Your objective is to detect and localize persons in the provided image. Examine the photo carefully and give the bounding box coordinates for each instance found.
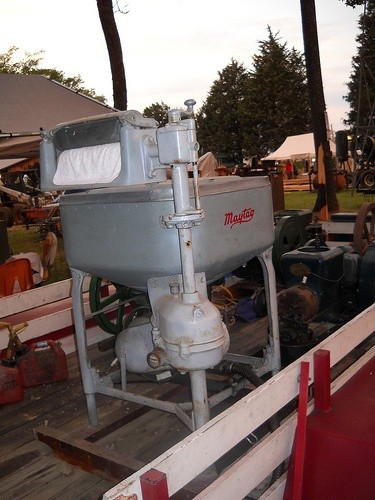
[285,157,311,179]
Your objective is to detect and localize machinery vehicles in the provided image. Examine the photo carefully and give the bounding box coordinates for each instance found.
[0,168,61,226]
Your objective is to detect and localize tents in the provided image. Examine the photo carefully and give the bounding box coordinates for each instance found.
[261,132,353,179]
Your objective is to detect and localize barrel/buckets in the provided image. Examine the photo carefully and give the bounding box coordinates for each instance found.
[0,321,69,405]
